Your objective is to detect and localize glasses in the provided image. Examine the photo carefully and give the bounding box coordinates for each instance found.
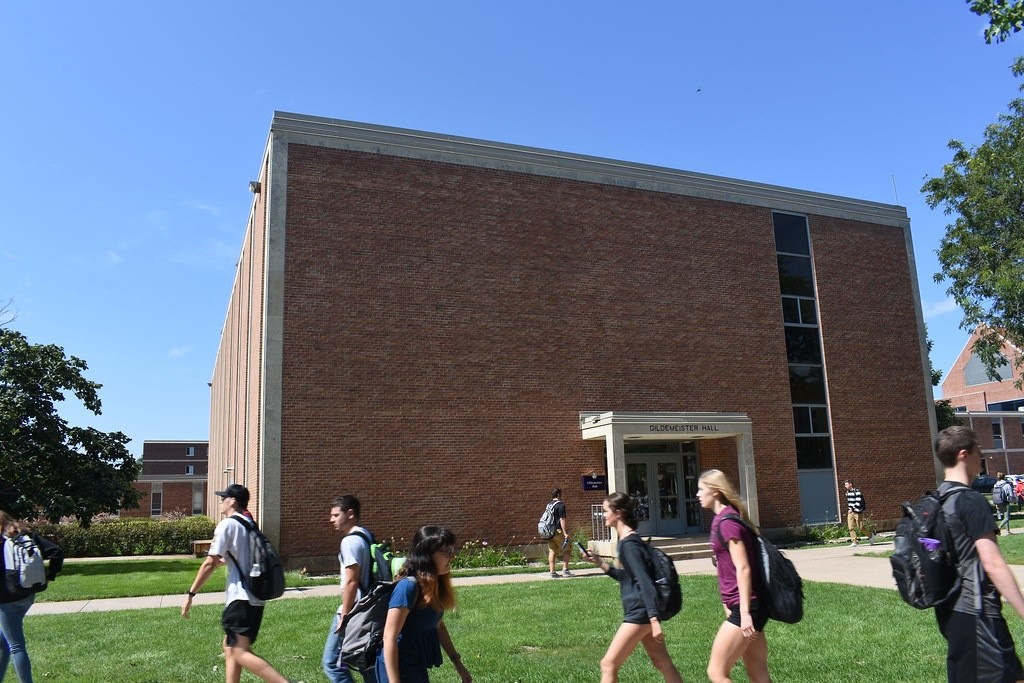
[437,548,454,557]
[221,496,227,500]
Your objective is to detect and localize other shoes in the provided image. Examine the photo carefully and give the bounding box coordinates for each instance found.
[870,532,874,545]
[1006,531,1011,534]
[849,542,857,547]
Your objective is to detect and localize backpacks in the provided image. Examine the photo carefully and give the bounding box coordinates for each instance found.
[2,530,48,593]
[616,538,683,620]
[226,515,285,600]
[340,531,408,593]
[333,576,422,672]
[717,513,803,624]
[992,482,1009,505]
[890,486,979,609]
[537,500,565,539]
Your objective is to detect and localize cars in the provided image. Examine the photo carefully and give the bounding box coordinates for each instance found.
[972,476,998,494]
[1004,474,1024,486]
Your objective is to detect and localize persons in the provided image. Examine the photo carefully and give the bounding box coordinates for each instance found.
[182,484,288,683]
[0,509,64,683]
[579,492,683,683]
[375,527,472,683]
[322,495,375,683]
[934,425,1024,683]
[697,469,773,683]
[1014,478,1024,512]
[546,488,576,577]
[844,479,874,548]
[994,471,1013,536]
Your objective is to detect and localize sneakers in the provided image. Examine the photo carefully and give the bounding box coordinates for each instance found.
[560,570,575,577]
[549,572,563,578]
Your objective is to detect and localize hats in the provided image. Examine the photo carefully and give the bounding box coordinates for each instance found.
[215,484,249,502]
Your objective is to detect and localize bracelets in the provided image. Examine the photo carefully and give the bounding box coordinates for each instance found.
[450,654,461,662]
[187,589,195,596]
